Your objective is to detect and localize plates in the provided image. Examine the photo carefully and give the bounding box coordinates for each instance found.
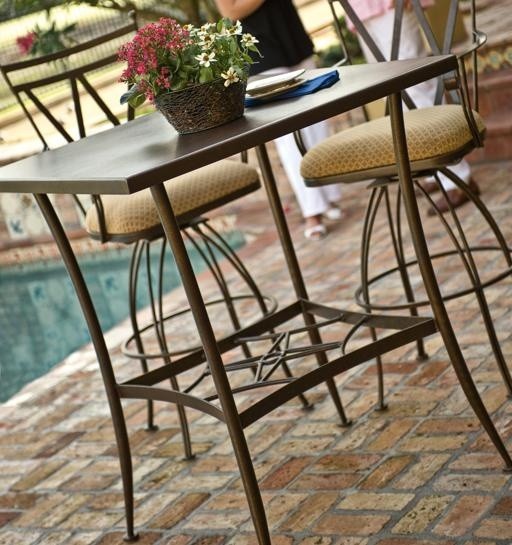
[246,68,308,103]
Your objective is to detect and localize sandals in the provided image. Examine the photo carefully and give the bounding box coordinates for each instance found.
[304,206,344,241]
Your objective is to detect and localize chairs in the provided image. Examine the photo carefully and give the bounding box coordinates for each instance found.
[0,8,315,461]
[294,0,511,412]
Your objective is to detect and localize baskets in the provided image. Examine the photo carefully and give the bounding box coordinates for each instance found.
[154,66,247,135]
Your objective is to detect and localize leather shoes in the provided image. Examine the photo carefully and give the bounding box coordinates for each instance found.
[415,177,480,216]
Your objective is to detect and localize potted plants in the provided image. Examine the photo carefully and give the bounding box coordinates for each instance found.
[115,15,264,135]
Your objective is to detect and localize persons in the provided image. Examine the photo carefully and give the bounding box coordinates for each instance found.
[345,0,481,216]
[213,0,348,240]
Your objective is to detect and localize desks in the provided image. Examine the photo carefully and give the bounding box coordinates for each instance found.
[2,52,512,545]
